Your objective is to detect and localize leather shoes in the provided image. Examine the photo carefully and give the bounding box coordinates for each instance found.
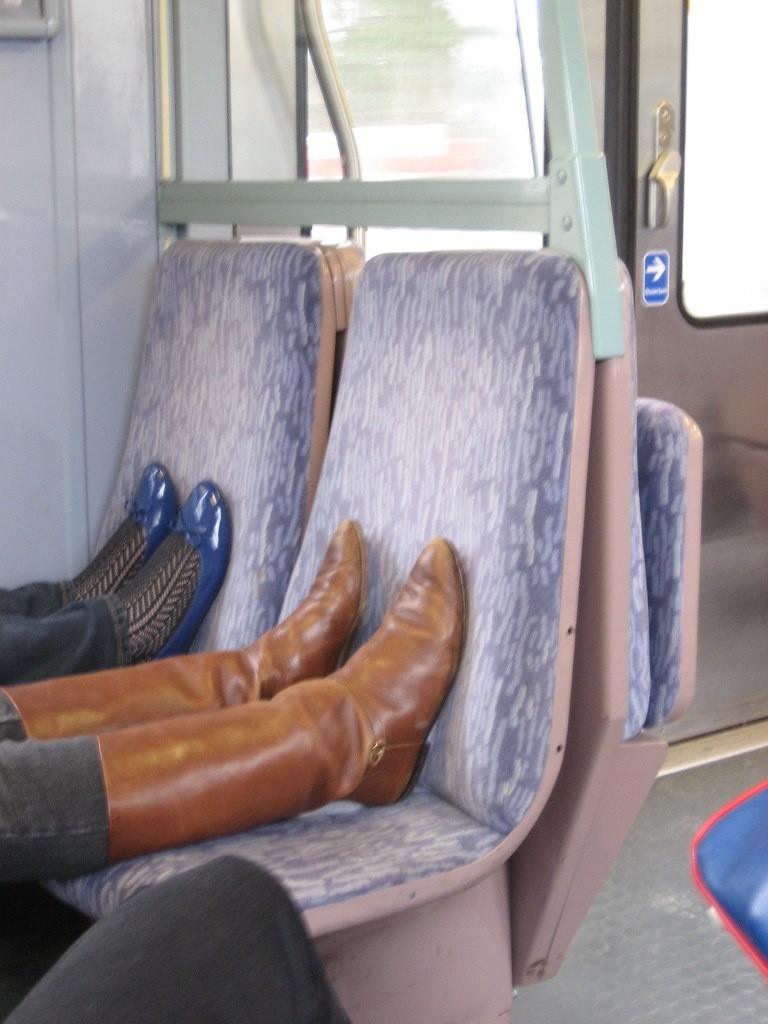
[122,461,179,568]
[155,481,233,660]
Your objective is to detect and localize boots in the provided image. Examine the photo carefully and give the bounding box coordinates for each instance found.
[0,520,367,745]
[97,537,468,866]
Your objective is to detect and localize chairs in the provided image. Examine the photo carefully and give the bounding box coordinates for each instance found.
[40,236,702,1024]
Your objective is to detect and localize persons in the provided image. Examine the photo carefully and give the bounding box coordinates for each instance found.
[0,518,466,884]
[0,463,232,688]
